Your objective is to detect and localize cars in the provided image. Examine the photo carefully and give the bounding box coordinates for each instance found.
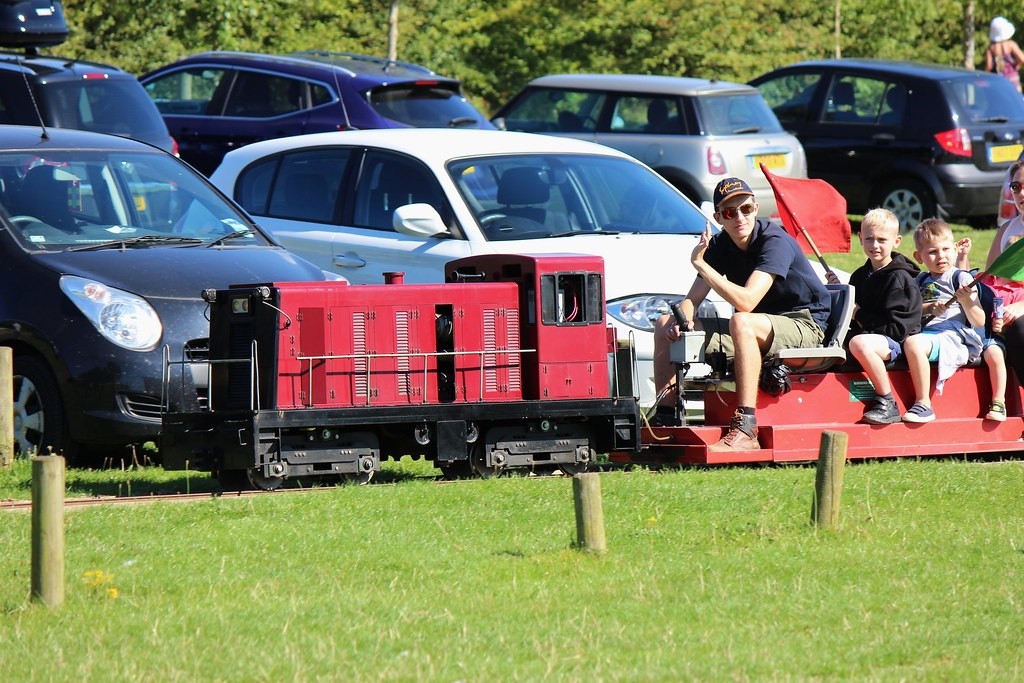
[742,60,1024,233]
[481,72,814,227]
[175,127,856,449]
[0,124,352,460]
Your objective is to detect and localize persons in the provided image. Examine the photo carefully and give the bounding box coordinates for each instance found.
[646,177,832,451]
[825,148,1024,423]
[987,16,1024,94]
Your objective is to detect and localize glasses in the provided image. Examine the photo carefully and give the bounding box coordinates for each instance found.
[1009,181,1024,194]
[718,203,754,220]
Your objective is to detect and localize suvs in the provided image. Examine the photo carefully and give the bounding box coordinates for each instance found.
[0,0,181,233]
[126,51,500,229]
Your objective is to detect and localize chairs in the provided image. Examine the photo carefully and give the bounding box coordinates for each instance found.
[643,102,669,132]
[368,161,428,230]
[281,173,333,223]
[769,283,853,370]
[497,168,556,223]
[876,87,907,126]
[830,81,858,122]
[16,164,78,232]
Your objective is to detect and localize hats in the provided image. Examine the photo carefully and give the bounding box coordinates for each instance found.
[990,17,1016,42]
[713,177,754,208]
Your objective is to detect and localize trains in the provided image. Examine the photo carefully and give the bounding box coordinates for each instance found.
[156,255,1020,492]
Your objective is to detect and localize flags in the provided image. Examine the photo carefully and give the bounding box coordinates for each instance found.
[761,163,852,254]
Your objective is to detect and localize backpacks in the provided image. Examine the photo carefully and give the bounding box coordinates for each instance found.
[919,269,999,339]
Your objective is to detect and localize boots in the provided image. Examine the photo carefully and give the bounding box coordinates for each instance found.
[645,412,682,426]
[708,408,761,451]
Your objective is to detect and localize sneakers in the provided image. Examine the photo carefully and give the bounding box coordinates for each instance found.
[862,396,901,423]
[986,399,1006,422]
[901,403,936,422]
